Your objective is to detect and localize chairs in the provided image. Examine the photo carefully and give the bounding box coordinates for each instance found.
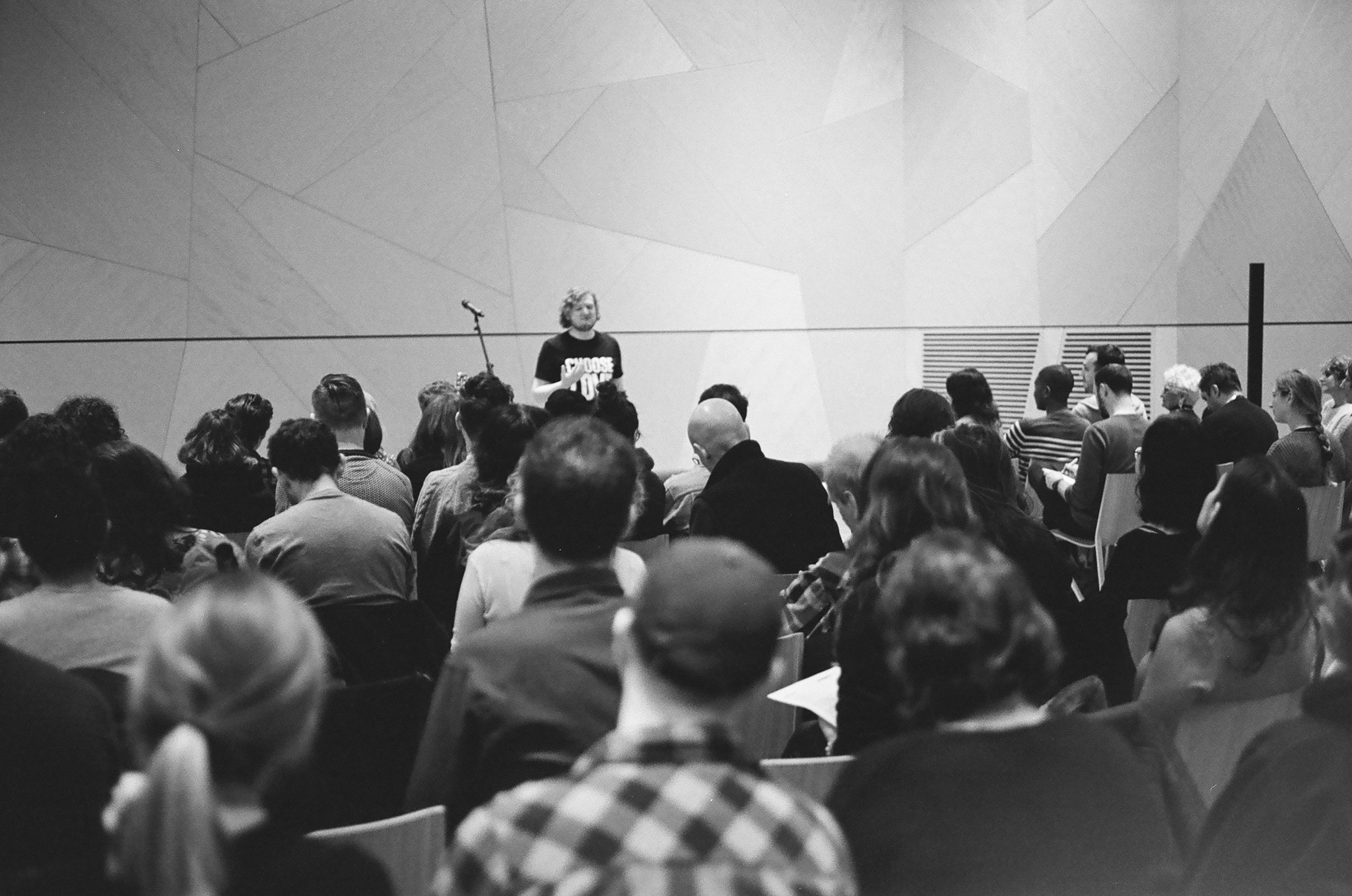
[1298,479,1347,568]
[1189,712,1352,896]
[1046,470,1149,592]
[293,593,424,802]
[733,633,804,763]
[758,753,858,804]
[613,534,668,578]
[1165,683,1311,815]
[305,804,450,894]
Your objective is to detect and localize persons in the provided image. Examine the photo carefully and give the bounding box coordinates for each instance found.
[801,345,1350,665]
[683,397,839,580]
[0,455,178,677]
[92,570,395,896]
[777,435,985,708]
[424,536,854,896]
[528,288,624,399]
[96,439,218,602]
[405,413,630,827]
[1133,455,1325,748]
[3,373,753,550]
[1174,523,1352,896]
[244,415,419,605]
[822,524,1184,896]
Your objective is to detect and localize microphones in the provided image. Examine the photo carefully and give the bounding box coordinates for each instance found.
[462,300,485,318]
